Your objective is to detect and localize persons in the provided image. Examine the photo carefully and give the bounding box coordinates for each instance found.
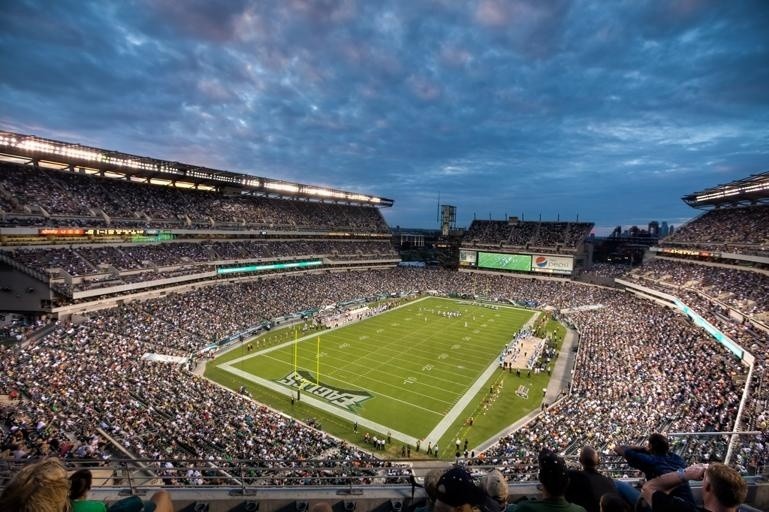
[0,161,769,512]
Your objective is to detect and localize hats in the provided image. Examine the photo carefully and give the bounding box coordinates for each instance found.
[424,461,510,510]
